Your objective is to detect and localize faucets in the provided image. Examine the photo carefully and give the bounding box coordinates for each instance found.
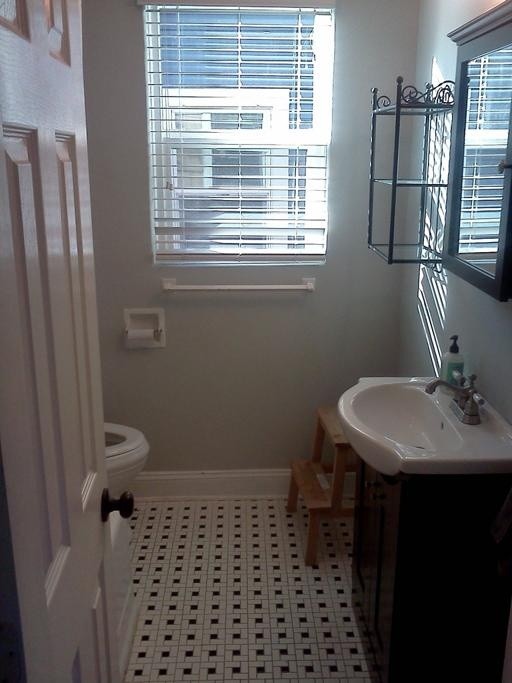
[423,378,468,396]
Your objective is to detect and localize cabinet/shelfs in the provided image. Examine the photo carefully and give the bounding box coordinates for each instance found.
[366,74,455,274]
[350,455,511,683]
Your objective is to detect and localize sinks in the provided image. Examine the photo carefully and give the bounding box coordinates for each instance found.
[335,375,512,473]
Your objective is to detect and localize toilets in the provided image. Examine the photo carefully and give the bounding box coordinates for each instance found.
[103,425,150,492]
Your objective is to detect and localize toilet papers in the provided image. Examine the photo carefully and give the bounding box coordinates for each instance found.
[126,329,155,341]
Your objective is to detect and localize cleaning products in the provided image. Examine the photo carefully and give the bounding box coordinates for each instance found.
[435,336,465,388]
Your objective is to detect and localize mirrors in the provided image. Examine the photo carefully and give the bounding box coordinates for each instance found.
[440,0,511,302]
